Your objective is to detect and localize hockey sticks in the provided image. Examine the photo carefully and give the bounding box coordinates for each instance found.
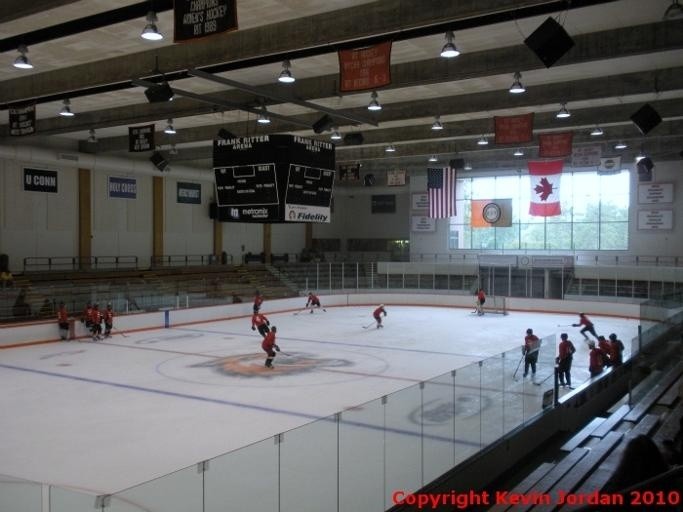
[533,372,553,386]
[513,354,525,382]
[362,314,384,329]
[470,308,477,313]
[113,325,130,338]
[293,306,308,316]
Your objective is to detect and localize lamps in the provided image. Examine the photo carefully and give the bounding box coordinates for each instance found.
[7,8,651,169]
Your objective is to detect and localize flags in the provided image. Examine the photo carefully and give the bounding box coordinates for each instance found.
[471,199,513,227]
[424,167,457,217]
[527,160,561,216]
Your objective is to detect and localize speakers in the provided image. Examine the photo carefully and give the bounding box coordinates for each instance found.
[629,104,662,136]
[144,84,174,103]
[639,157,654,173]
[344,134,364,145]
[523,17,574,68]
[312,114,333,134]
[449,159,464,168]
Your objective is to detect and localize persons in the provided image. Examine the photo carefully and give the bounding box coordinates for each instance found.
[251,308,279,354]
[372,304,388,329]
[607,333,624,367]
[586,341,609,378]
[0,270,13,287]
[522,329,539,379]
[572,312,600,340]
[475,288,486,315]
[596,336,612,366]
[305,292,327,314]
[12,289,113,342]
[261,326,278,368]
[555,333,576,386]
[252,290,264,313]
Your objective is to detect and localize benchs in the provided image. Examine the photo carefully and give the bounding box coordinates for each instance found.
[398,269,682,303]
[0,250,396,327]
[475,345,682,512]
